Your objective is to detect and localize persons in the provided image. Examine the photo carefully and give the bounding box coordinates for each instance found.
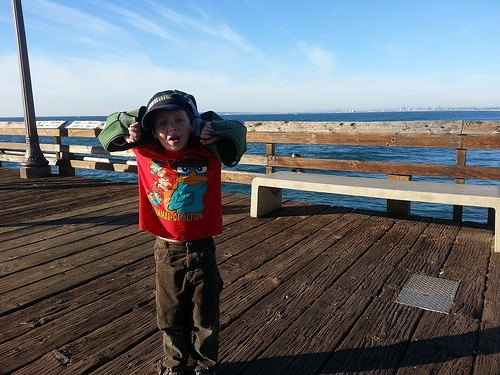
[291,152,305,173]
[99,91,249,375]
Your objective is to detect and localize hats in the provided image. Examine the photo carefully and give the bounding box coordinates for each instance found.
[141,91,188,130]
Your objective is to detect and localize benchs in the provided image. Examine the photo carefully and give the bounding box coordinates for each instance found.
[250,171,499,251]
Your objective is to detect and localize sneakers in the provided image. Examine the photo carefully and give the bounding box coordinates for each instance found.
[195,368,216,375]
[161,367,184,375]
